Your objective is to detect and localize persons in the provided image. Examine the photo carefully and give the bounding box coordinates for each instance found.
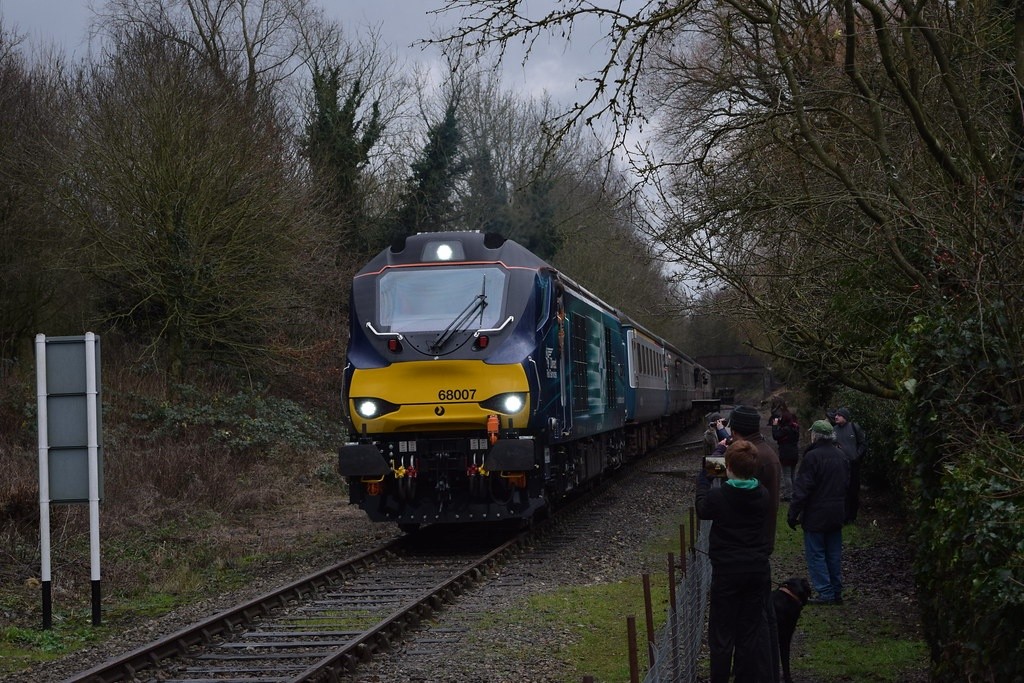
[788,420,852,607]
[709,407,783,602]
[789,413,798,428]
[823,409,838,426]
[832,409,867,523]
[699,408,733,461]
[769,409,799,501]
[692,439,778,683]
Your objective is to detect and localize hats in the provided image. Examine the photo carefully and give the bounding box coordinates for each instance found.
[808,420,833,434]
[728,406,761,432]
[834,408,851,419]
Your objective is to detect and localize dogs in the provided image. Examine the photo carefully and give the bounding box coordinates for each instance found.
[771,577,811,683]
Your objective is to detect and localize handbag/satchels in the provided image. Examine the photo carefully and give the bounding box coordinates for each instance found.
[861,449,867,462]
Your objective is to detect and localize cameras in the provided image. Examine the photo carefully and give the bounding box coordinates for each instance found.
[709,418,727,428]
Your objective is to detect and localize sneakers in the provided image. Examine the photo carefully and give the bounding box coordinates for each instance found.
[808,592,832,605]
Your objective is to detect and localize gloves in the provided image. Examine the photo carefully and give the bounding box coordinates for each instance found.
[699,469,716,488]
[787,517,800,531]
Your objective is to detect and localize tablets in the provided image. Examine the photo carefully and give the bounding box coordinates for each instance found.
[703,455,733,480]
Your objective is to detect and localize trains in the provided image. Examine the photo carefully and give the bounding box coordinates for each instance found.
[717,385,736,403]
[336,229,717,546]
